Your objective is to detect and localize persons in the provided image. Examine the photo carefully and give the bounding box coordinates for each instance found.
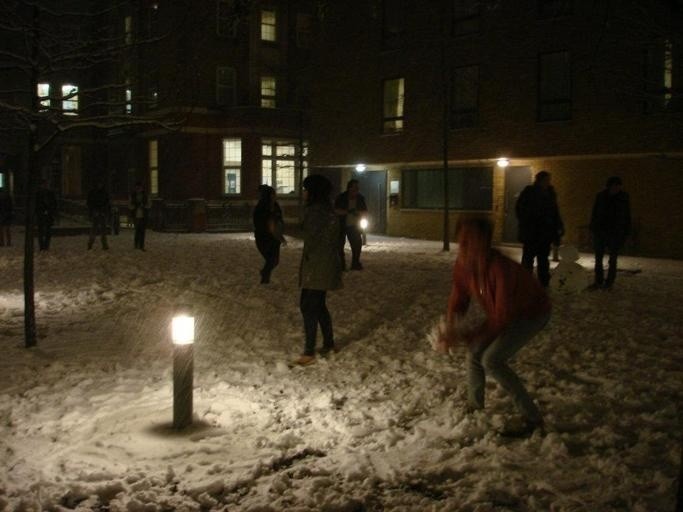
[128,180,153,252]
[0,186,12,250]
[584,176,632,292]
[84,176,110,252]
[252,183,288,284]
[34,177,55,252]
[271,174,345,365]
[514,169,565,287]
[336,180,369,271]
[430,213,551,440]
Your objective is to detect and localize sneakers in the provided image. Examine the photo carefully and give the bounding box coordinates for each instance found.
[497,421,544,439]
[297,353,316,366]
